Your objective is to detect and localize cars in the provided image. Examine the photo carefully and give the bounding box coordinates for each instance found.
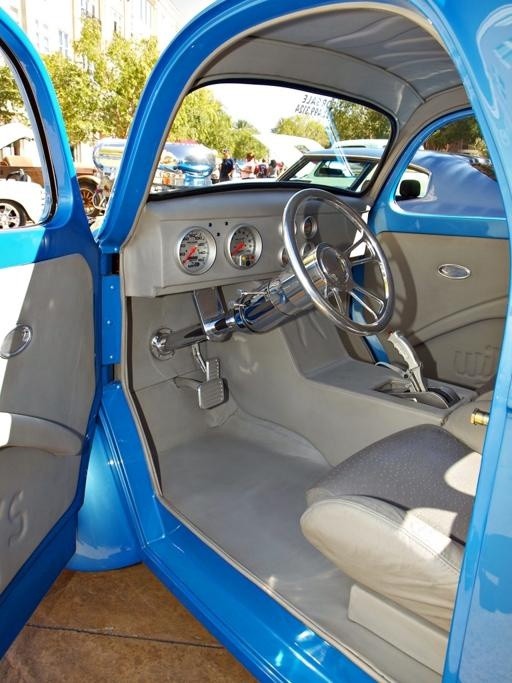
[0,122,45,228]
[296,137,427,199]
[2,0,510,683]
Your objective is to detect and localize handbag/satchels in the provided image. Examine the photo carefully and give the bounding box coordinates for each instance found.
[254,166,260,175]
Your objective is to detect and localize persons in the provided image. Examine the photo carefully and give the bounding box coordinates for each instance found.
[218,147,236,183]
[256,158,270,177]
[266,158,278,178]
[210,149,223,184]
[238,151,259,178]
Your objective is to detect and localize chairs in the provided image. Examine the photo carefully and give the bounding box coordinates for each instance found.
[299,400,500,676]
